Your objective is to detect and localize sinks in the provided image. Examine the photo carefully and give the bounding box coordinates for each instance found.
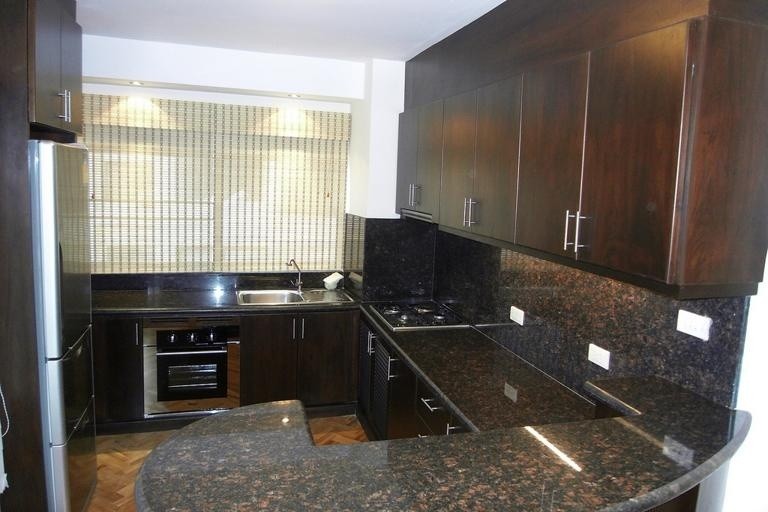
[236,289,305,306]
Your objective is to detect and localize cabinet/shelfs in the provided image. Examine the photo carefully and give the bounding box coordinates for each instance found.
[441,75,523,245]
[91,313,145,421]
[385,352,414,441]
[517,17,768,286]
[417,378,453,439]
[394,99,439,224]
[241,309,355,403]
[357,322,393,440]
[2,0,85,140]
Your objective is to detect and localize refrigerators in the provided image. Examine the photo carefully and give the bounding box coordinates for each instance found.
[29,138,98,512]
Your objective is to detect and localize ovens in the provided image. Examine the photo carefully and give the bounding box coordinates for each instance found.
[142,319,242,419]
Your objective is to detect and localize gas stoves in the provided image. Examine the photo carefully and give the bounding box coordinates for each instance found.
[369,299,470,330]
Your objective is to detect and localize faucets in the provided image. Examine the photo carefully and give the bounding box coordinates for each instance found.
[285,258,304,288]
